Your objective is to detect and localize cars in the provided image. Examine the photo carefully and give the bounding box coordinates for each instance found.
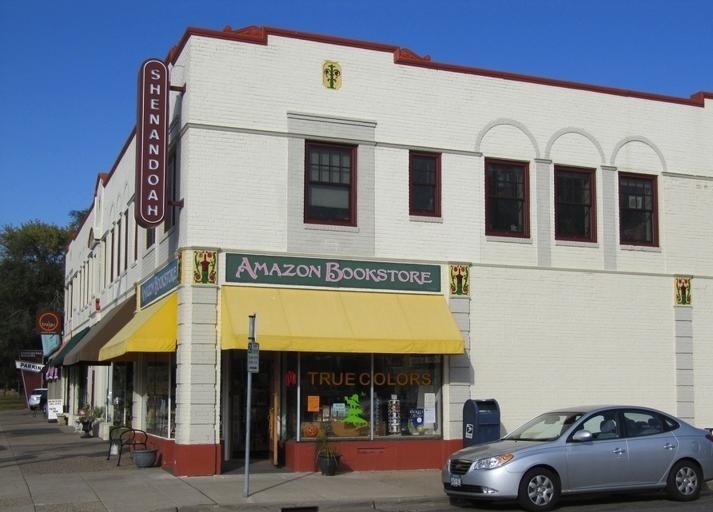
[317,395,423,421]
[442,399,712,512]
[28,388,48,410]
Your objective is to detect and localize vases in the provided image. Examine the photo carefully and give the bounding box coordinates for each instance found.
[56,412,65,425]
[133,447,157,467]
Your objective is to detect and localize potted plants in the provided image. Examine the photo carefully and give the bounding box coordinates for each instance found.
[75,401,103,438]
[313,424,343,476]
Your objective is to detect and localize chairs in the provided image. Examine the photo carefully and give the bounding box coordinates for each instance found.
[115,428,148,466]
[107,425,135,460]
